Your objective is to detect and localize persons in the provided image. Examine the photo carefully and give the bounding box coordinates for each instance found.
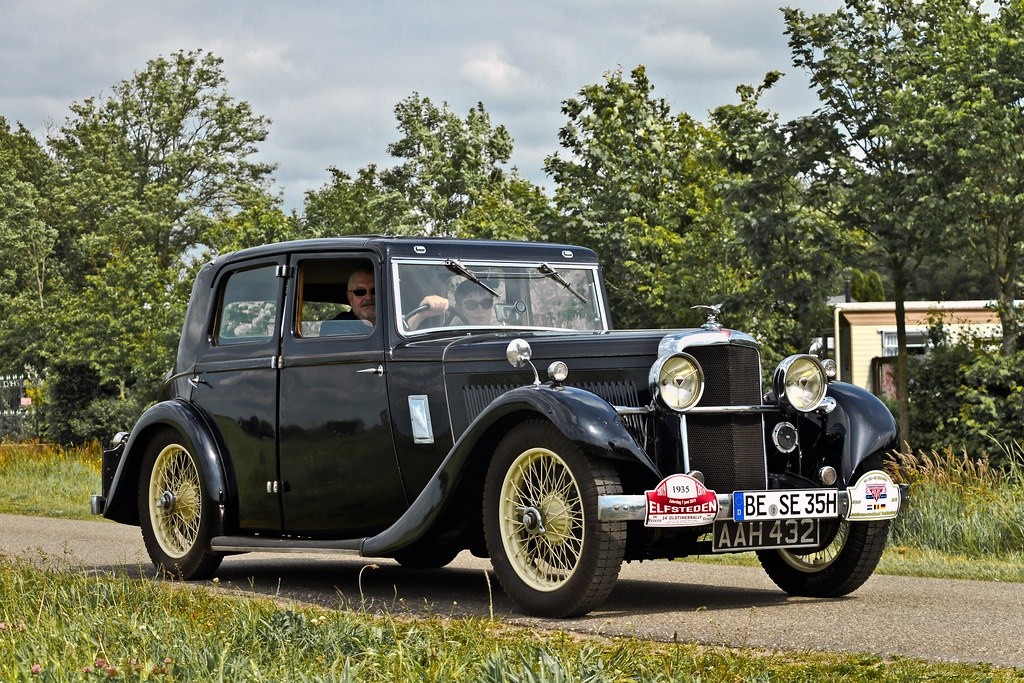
[318,270,449,337]
[455,279,495,325]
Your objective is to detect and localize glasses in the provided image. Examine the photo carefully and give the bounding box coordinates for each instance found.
[348,286,375,296]
[459,297,493,311]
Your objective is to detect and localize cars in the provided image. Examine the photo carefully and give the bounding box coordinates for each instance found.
[88,233,912,622]
